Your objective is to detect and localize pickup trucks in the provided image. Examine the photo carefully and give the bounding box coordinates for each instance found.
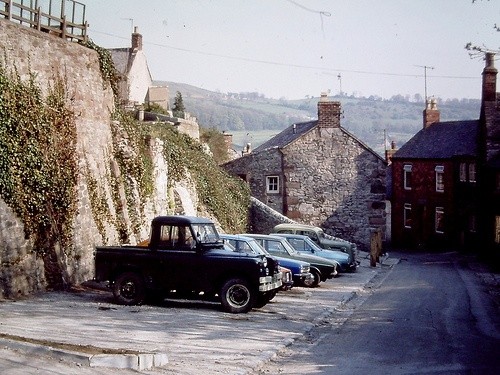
[94,215,284,314]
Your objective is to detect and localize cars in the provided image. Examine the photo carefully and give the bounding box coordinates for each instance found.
[200,234,311,290]
[268,233,350,278]
[238,234,337,288]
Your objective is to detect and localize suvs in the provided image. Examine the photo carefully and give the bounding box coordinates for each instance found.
[272,223,361,273]
